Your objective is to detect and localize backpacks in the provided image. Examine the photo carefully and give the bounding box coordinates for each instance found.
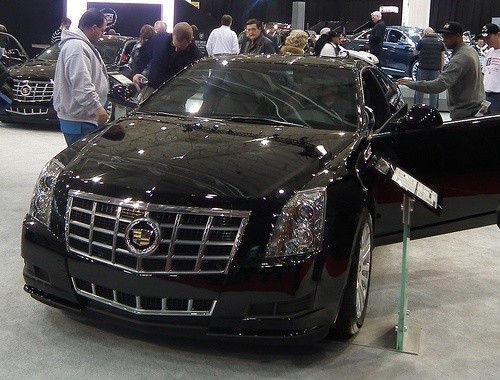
[314,36,335,56]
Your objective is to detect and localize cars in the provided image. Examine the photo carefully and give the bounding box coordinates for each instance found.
[349,25,450,82]
[0,31,138,128]
[20,52,499,349]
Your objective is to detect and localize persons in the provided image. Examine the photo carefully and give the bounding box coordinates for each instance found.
[52,7,111,147]
[394,21,485,121]
[470,23,500,114]
[0,10,500,108]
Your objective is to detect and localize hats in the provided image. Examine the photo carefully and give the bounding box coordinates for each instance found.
[478,24,500,37]
[437,22,465,34]
[328,31,339,37]
[320,27,331,36]
